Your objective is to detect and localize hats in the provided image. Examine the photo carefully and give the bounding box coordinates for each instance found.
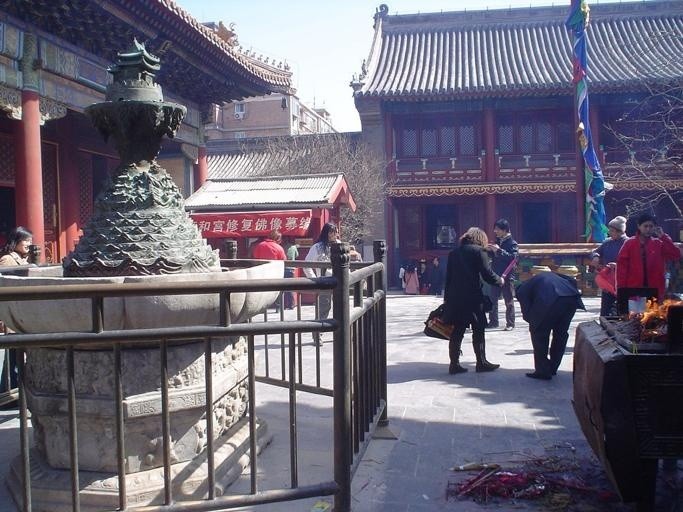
[607,216,628,233]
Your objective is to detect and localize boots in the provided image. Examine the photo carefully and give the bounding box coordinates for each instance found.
[447,343,468,374]
[473,341,500,373]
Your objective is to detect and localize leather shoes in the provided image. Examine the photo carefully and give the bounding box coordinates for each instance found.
[487,324,498,328]
[524,370,557,381]
[505,325,514,330]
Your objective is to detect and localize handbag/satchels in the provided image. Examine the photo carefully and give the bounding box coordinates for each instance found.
[423,303,458,340]
[596,262,618,297]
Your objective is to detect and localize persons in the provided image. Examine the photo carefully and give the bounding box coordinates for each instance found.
[252,231,288,261]
[485,219,519,331]
[282,237,299,310]
[443,226,505,374]
[302,222,340,346]
[350,245,366,297]
[398,256,443,297]
[590,214,683,317]
[1,225,33,407]
[514,273,586,381]
